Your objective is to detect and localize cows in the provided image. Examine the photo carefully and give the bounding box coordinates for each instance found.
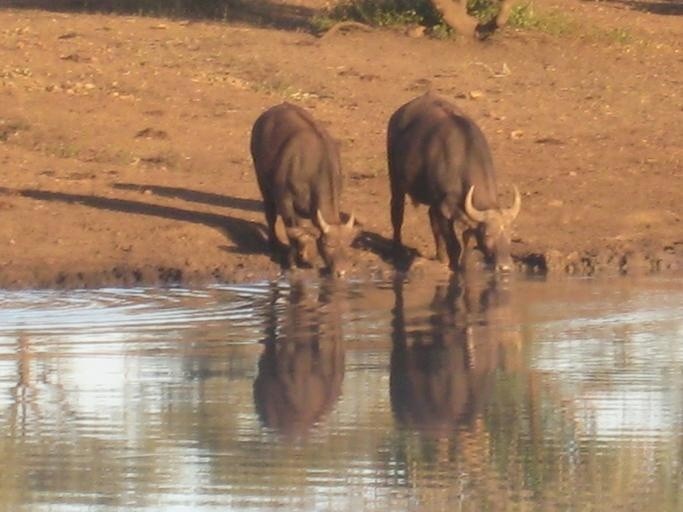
[249,101,355,280]
[386,92,520,275]
